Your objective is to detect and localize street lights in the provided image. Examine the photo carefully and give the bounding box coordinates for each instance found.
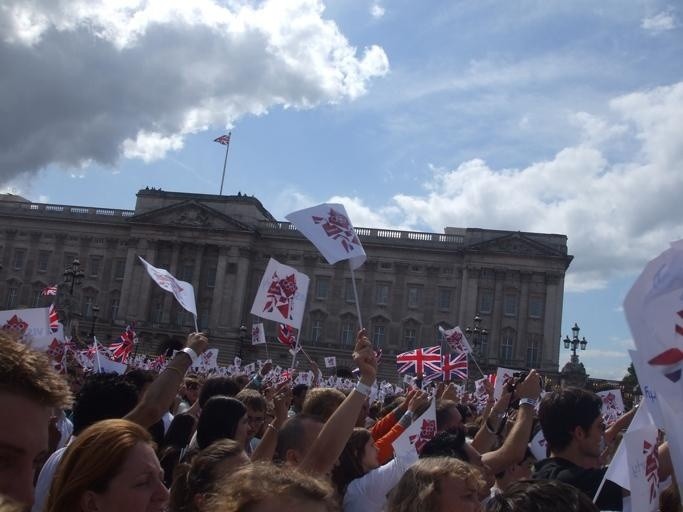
[465,312,487,377]
[62,257,84,296]
[89,303,100,337]
[562,321,587,356]
[237,319,247,362]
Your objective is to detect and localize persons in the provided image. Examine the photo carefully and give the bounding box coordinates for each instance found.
[1,323,681,512]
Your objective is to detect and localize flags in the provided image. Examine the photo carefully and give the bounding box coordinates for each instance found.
[40,284,57,296]
[47,302,63,312]
[250,258,312,331]
[623,238,683,416]
[285,202,367,273]
[139,257,199,321]
[214,135,231,145]
[47,305,59,329]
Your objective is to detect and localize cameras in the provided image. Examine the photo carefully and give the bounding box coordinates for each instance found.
[507,371,544,410]
